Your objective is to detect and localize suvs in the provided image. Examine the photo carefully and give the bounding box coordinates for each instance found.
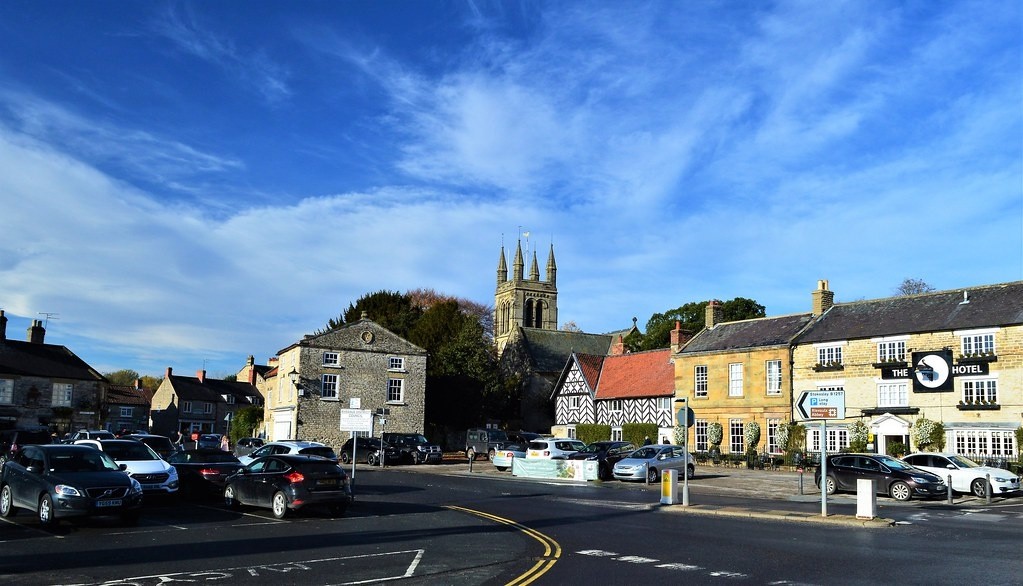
[817,453,947,503]
[380,431,442,465]
[466,428,524,463]
[568,441,636,481]
[527,438,589,461]
[236,441,338,469]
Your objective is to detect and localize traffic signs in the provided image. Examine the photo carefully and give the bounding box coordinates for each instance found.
[795,390,846,421]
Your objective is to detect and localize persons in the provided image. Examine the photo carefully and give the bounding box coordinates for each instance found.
[3,427,236,465]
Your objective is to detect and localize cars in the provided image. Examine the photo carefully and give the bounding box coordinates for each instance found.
[492,434,542,471]
[898,452,1020,499]
[1,424,248,527]
[224,453,352,519]
[613,444,695,485]
[340,436,400,466]
